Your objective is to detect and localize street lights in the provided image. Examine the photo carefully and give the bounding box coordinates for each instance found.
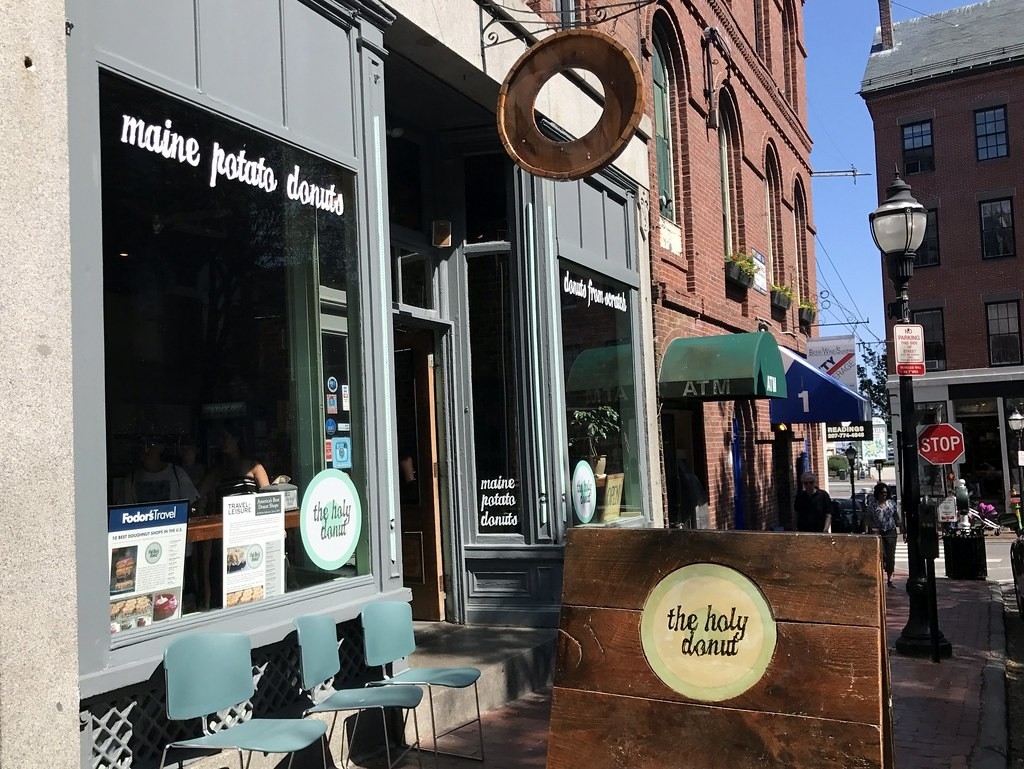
[845,443,857,532]
[1008,406,1024,526]
[874,459,884,483]
[868,163,953,652]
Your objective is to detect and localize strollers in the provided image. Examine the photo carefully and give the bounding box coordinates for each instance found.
[968,502,1002,536]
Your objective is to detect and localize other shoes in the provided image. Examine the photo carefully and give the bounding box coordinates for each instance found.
[887,581,896,588]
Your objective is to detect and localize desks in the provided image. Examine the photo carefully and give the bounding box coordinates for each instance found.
[184,510,299,610]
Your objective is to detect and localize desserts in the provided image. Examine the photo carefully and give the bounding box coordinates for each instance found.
[227,547,246,572]
[110,558,177,634]
[227,586,263,606]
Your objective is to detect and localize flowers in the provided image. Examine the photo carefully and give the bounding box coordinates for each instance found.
[798,302,817,312]
[724,252,760,278]
[773,284,796,306]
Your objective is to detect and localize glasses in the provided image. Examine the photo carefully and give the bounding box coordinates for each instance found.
[802,481,813,486]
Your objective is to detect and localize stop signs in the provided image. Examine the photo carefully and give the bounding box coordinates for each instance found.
[917,423,966,465]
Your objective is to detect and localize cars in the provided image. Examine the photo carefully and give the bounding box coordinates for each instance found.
[832,498,866,529]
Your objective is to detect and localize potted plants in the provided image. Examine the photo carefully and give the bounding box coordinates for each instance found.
[568,406,621,478]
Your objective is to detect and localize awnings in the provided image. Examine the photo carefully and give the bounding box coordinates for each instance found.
[768,346,872,424]
[659,331,790,400]
[568,345,633,405]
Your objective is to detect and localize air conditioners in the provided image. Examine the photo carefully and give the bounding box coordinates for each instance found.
[926,361,946,371]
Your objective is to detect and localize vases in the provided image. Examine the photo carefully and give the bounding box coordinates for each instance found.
[799,308,812,323]
[771,291,791,310]
[725,262,753,288]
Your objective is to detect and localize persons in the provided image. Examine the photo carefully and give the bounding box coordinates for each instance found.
[862,483,901,588]
[792,471,833,533]
[109,425,271,612]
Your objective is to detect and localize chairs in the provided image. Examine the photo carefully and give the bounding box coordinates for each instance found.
[290,614,423,769]
[160,634,328,769]
[345,602,484,769]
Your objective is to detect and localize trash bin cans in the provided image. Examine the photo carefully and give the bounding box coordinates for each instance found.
[941,524,987,581]
